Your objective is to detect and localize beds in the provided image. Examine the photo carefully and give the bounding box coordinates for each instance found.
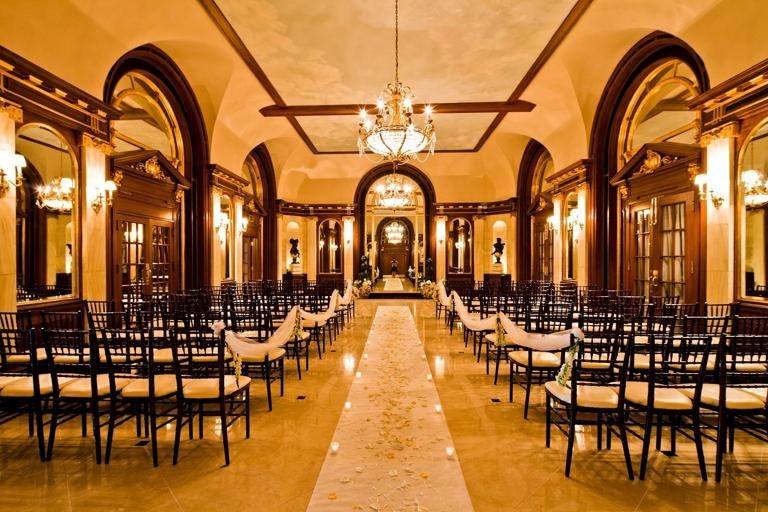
[2,276,357,383]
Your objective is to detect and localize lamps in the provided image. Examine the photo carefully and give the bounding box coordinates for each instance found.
[214,213,228,232]
[567,212,584,243]
[545,215,559,235]
[0,149,26,196]
[691,171,725,209]
[89,180,116,215]
[355,0,438,172]
[740,170,766,207]
[368,173,417,213]
[237,217,247,236]
[33,142,73,213]
[383,222,405,244]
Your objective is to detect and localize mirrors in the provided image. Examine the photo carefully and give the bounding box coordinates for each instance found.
[14,111,80,305]
[353,162,437,299]
[559,192,576,279]
[375,217,414,279]
[445,215,472,274]
[317,216,344,275]
[734,112,766,308]
[217,192,236,281]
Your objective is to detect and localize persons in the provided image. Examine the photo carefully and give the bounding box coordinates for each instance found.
[390,257,400,278]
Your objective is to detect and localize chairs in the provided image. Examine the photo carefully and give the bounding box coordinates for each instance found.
[432,278,763,388]
[608,334,713,483]
[670,334,766,485]
[99,326,191,469]
[223,310,287,412]
[167,325,253,466]
[1,373,35,438]
[39,326,138,465]
[1,328,82,462]
[508,314,573,420]
[543,332,637,481]
